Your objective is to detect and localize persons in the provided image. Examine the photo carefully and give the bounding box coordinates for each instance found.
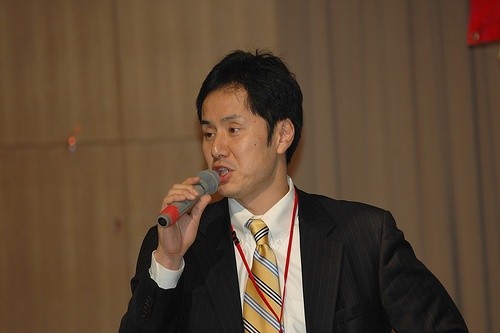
[118,47,469,333]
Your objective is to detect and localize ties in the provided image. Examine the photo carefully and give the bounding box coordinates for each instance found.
[242,219,285,333]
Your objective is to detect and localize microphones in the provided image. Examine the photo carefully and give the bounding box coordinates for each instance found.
[157,169,221,228]
